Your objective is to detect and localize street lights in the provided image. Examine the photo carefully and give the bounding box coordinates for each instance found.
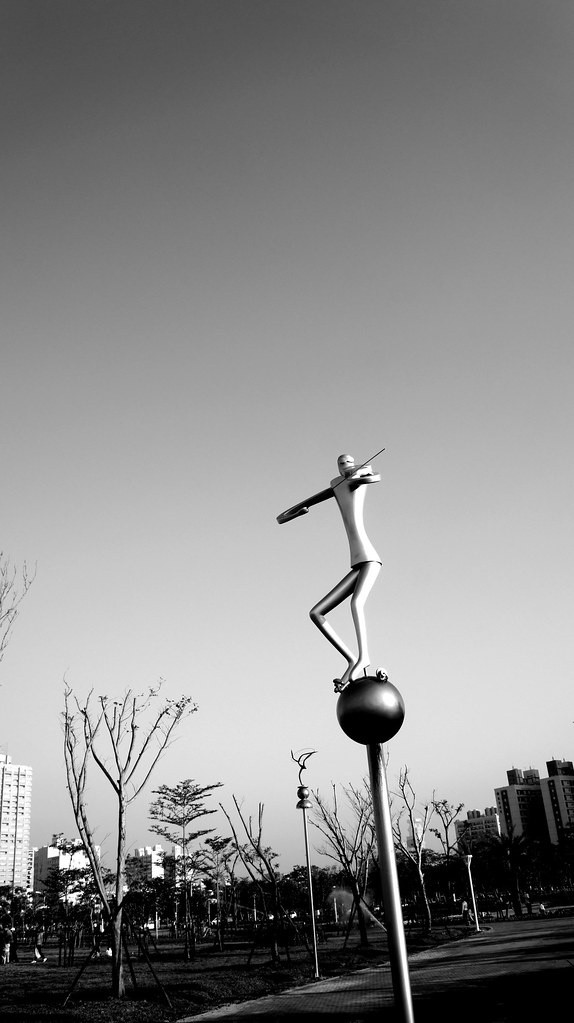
[463,854,481,931]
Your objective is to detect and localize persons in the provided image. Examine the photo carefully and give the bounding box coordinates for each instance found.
[31,927,47,964]
[0,925,19,964]
[524,890,533,915]
[461,898,470,926]
[539,902,545,914]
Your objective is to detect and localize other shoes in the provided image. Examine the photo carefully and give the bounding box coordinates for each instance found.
[31,960,36,964]
[42,958,47,962]
